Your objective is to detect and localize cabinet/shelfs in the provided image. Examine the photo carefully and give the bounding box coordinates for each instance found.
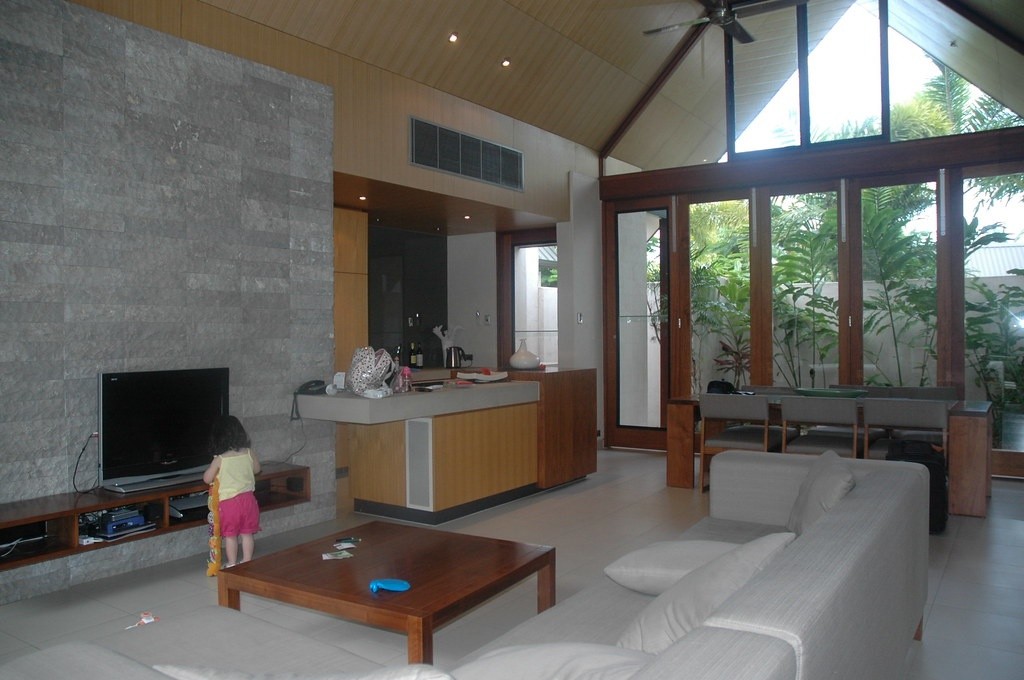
[0,460,311,573]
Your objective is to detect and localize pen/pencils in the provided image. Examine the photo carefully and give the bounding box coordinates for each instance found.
[336,537,362,542]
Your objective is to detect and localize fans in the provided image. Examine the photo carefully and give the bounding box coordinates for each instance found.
[643,0,777,43]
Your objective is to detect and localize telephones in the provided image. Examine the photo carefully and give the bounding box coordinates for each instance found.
[297,379,328,395]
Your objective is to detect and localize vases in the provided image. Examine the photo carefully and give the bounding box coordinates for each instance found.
[509,339,539,370]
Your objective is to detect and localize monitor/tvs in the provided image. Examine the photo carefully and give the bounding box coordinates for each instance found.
[98,366,229,493]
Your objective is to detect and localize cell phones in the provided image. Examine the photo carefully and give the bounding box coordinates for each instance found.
[336,536,362,543]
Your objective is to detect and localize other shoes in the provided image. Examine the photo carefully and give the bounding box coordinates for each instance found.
[222,561,243,569]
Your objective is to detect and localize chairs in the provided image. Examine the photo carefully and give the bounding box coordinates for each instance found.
[888,386,958,442]
[779,394,863,459]
[726,385,802,438]
[806,385,891,442]
[863,398,949,469]
[697,392,782,495]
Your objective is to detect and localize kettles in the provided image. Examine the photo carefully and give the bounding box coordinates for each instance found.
[446,346,466,368]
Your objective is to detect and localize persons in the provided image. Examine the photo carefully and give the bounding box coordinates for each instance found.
[203,416,262,571]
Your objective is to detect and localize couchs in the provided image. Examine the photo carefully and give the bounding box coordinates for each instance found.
[362,449,931,680]
[0,602,386,680]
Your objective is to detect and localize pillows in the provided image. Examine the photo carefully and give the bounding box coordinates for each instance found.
[615,531,796,655]
[450,641,656,680]
[604,540,741,596]
[785,450,856,536]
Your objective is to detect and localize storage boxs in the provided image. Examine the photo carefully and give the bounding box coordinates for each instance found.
[665,392,994,517]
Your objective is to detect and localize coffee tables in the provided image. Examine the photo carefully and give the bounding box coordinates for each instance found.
[216,519,556,666]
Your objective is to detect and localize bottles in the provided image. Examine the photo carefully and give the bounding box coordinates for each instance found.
[416,342,423,369]
[509,339,540,370]
[393,345,400,367]
[409,342,416,368]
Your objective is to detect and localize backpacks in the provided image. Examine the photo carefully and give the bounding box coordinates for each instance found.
[351,346,399,395]
